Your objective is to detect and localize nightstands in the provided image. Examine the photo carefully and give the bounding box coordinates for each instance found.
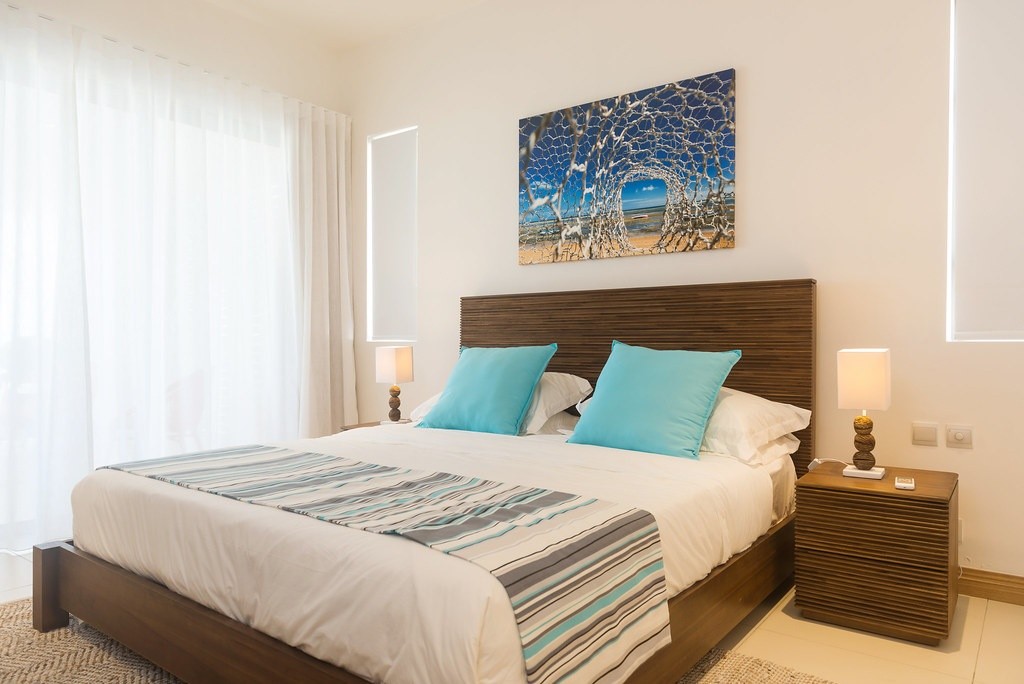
[340,421,402,430]
[794,462,959,648]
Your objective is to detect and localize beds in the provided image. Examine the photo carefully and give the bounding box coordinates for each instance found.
[26,278,817,684]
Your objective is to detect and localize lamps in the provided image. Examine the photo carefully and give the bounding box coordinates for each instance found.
[375,345,412,425]
[837,348,891,479]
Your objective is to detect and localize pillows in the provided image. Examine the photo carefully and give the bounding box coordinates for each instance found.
[565,339,742,458]
[576,387,812,461]
[742,434,801,466]
[414,343,558,434]
[410,371,593,436]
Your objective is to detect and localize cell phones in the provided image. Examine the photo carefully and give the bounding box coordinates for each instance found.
[894,477,915,490]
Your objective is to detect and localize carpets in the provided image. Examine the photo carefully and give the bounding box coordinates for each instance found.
[0,598,833,684]
